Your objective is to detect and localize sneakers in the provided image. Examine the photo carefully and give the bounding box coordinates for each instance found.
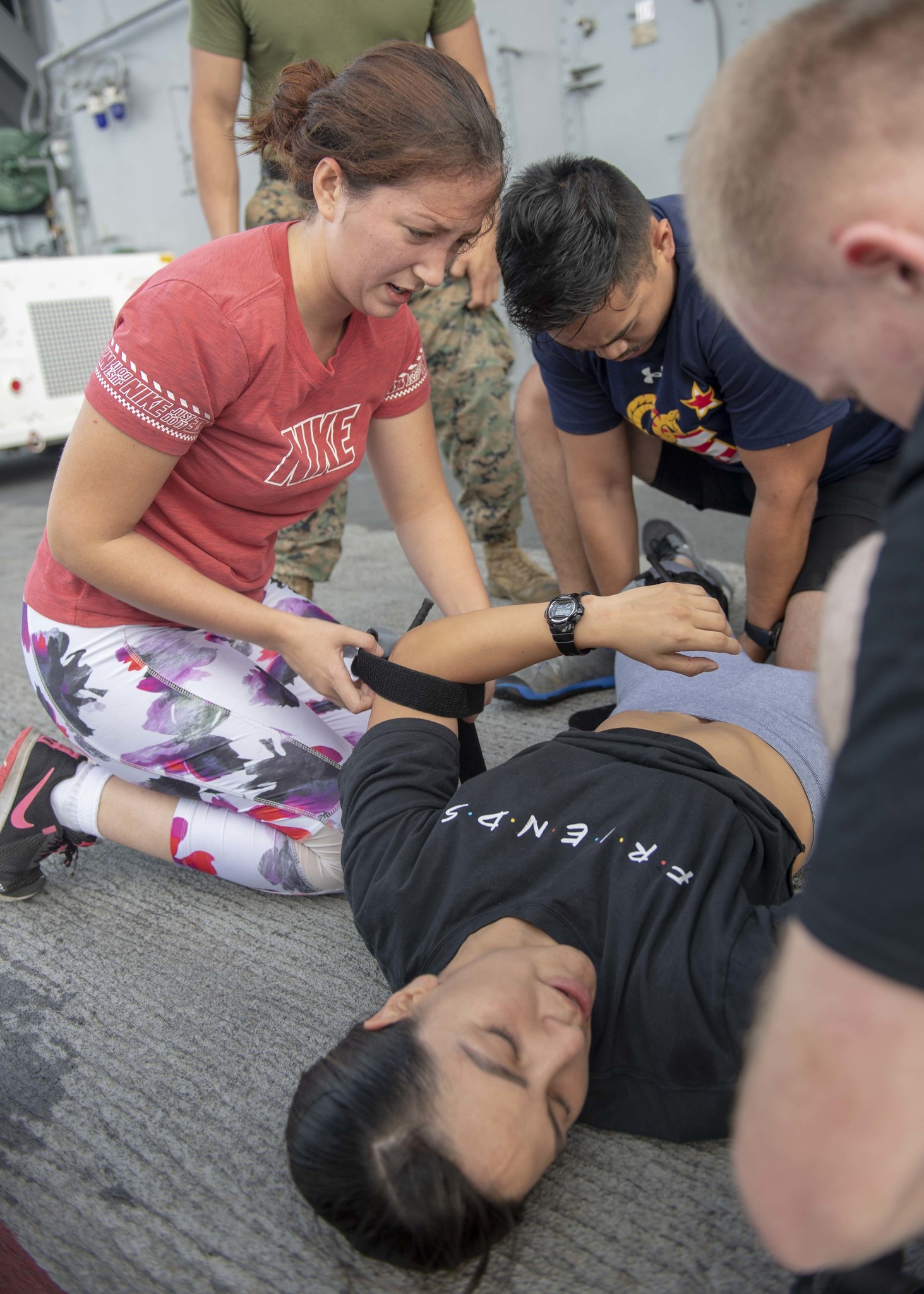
[643,519,735,603]
[0,727,97,902]
[493,645,615,706]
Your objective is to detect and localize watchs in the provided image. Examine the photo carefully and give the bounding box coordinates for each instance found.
[544,590,599,656]
[744,619,784,651]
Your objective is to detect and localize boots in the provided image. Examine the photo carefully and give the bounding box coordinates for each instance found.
[483,529,559,605]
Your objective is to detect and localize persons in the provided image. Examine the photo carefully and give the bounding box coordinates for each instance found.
[495,151,902,703]
[0,42,497,901]
[189,1,564,605]
[679,0,924,1274]
[284,519,836,1272]
